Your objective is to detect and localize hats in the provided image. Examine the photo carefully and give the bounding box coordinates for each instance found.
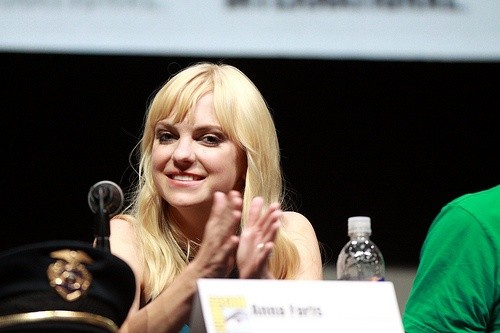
[0,241,137,333]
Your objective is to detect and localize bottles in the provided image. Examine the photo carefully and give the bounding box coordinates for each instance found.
[336,216,385,281]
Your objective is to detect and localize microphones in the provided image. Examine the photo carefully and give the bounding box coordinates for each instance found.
[88,180,124,251]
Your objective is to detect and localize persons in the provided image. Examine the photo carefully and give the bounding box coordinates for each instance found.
[402,185,500,333]
[110,64,322,333]
